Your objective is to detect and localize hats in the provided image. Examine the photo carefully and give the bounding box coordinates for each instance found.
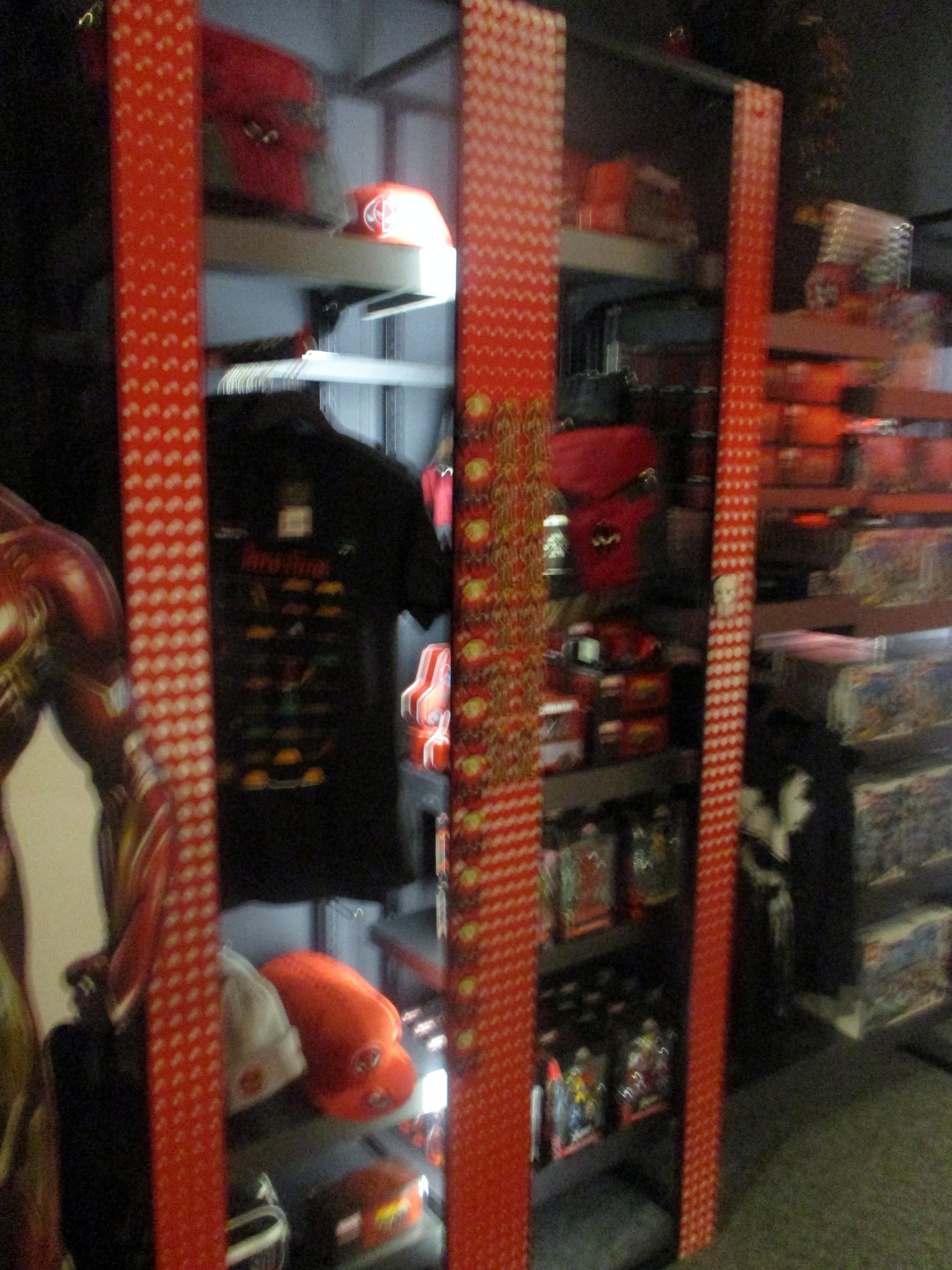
[349,180,455,245]
[222,947,305,1118]
[257,953,416,1122]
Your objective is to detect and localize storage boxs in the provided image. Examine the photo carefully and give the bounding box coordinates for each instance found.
[388,148,951,1165]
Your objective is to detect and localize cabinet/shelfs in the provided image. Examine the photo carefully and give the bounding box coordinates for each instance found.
[377,216,728,1263]
[602,305,952,1109]
[197,200,446,1270]
